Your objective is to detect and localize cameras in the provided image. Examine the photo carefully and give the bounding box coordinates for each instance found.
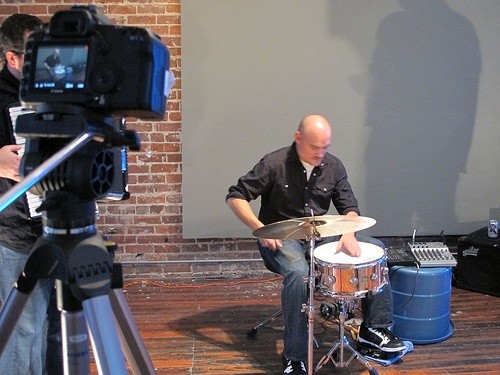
[19,4,170,121]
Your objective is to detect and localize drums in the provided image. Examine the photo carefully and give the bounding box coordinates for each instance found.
[311,240,388,299]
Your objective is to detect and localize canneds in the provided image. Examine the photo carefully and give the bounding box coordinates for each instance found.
[488,219,498,237]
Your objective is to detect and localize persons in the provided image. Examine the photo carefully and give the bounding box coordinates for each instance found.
[43,48,66,88]
[0,13,51,375]
[226,114,406,375]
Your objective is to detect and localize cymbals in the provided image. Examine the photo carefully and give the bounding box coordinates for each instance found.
[252,214,377,240]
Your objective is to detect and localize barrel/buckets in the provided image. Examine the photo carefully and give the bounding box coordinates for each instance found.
[387,266,455,343]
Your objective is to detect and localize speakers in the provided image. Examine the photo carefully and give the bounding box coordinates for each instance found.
[453,226,500,298]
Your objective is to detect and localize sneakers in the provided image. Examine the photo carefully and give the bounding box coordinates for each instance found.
[283,358,308,375]
[357,322,407,353]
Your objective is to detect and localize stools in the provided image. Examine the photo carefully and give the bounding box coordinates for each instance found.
[248,239,326,350]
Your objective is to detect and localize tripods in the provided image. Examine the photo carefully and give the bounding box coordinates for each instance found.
[0,104,157,375]
[313,302,380,375]
